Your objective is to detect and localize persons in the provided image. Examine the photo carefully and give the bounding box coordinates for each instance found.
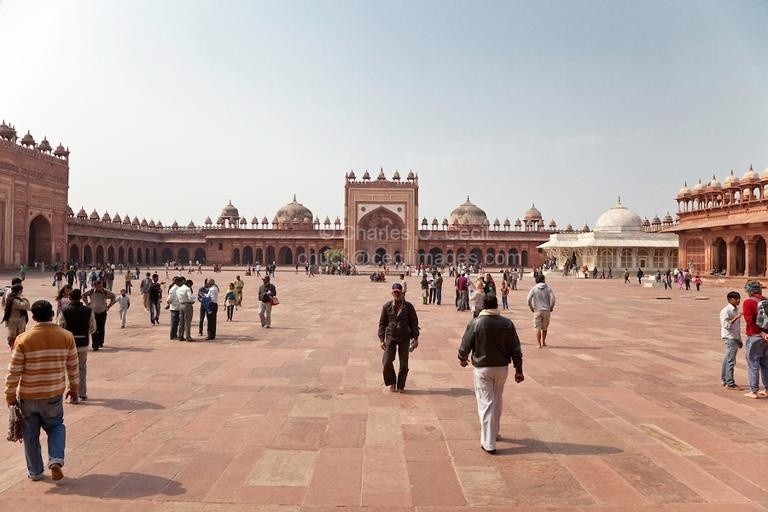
[4,300,83,481]
[741,280,768,401]
[719,290,745,390]
[223,282,240,322]
[56,288,98,404]
[0,245,734,349]
[457,294,527,454]
[1,283,30,351]
[525,273,557,348]
[377,282,422,395]
[257,274,277,329]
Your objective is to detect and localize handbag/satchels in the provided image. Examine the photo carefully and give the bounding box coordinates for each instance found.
[270,296,279,305]
[52,281,56,286]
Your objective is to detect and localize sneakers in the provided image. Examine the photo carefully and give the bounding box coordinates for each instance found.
[67,399,78,403]
[397,389,406,393]
[721,384,743,391]
[391,384,396,392]
[80,397,87,401]
[51,464,64,480]
[482,446,496,454]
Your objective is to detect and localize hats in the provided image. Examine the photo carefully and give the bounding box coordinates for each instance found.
[260,275,270,280]
[392,283,402,292]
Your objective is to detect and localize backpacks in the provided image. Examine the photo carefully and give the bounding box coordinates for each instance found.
[747,296,768,329]
